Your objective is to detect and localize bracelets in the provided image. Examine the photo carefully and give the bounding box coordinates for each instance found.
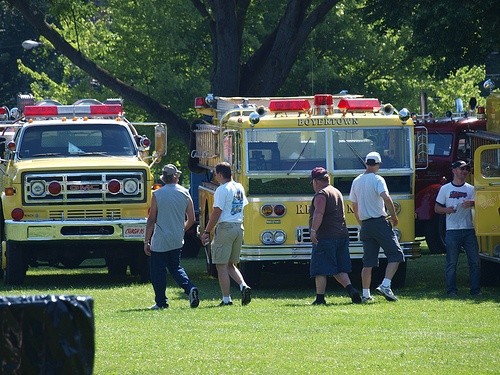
[311,228,317,232]
[204,231,210,234]
[145,240,151,244]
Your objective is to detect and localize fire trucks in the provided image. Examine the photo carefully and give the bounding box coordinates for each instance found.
[466,87,500,284]
[413,98,486,251]
[193,90,429,288]
[0,98,168,289]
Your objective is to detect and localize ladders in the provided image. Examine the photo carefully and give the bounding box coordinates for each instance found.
[194,129,219,159]
[465,131,500,170]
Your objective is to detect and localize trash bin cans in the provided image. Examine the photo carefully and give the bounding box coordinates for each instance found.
[0,295,95,375]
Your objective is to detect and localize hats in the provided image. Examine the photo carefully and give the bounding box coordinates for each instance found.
[365,152,383,165]
[163,164,183,176]
[309,167,327,184]
[450,160,470,168]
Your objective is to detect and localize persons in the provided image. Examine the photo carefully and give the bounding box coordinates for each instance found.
[434,160,487,298]
[308,167,362,305]
[201,163,253,307]
[349,151,405,301]
[144,163,199,309]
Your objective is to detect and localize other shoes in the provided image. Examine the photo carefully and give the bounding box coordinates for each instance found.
[187,287,199,308]
[470,291,487,296]
[146,302,168,310]
[218,300,232,306]
[375,285,398,301]
[447,288,458,297]
[311,299,326,305]
[241,285,251,306]
[351,289,375,304]
